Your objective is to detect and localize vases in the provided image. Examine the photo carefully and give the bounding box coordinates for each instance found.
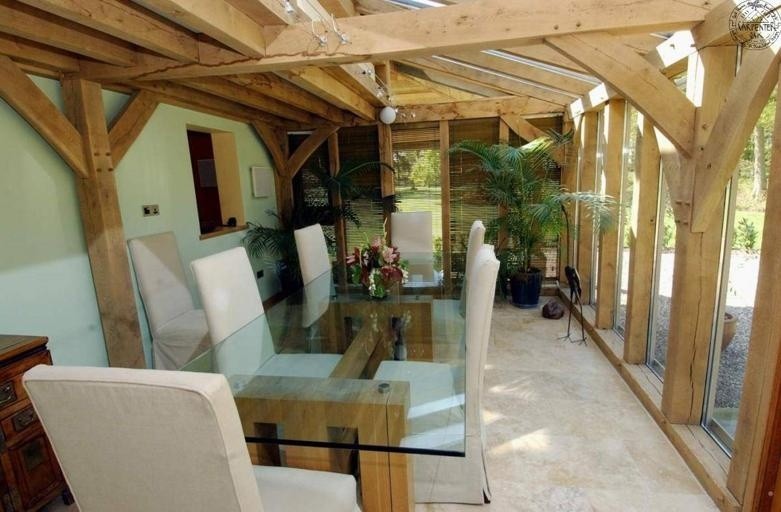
[722,311,737,352]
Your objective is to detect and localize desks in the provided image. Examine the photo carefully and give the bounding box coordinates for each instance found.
[178,250,466,512]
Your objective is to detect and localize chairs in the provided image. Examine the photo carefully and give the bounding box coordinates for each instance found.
[369,243,504,508]
[189,246,344,379]
[126,230,212,370]
[292,223,333,286]
[20,362,363,512]
[431,219,489,343]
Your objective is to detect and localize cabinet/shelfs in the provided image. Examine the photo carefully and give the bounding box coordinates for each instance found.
[1,331,76,512]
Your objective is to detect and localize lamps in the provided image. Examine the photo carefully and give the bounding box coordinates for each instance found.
[380,107,397,125]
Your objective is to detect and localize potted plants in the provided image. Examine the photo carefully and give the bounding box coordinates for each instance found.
[241,126,403,297]
[449,131,623,310]
